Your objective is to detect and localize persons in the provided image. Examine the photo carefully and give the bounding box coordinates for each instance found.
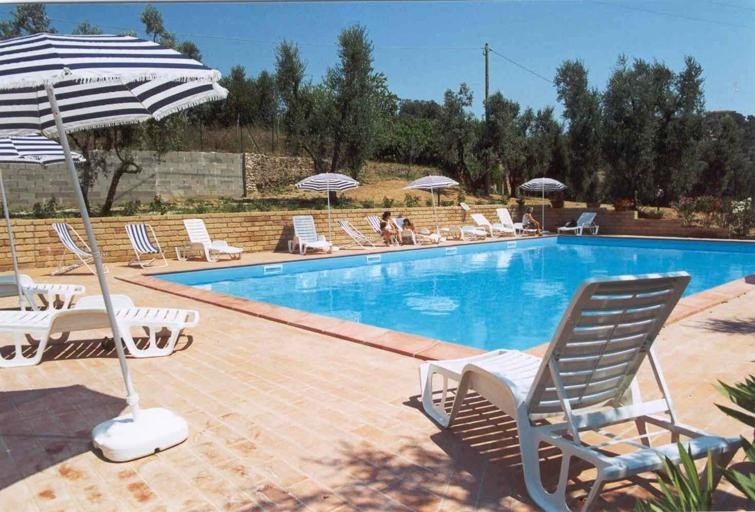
[378,211,404,245]
[519,205,543,236]
[403,218,442,245]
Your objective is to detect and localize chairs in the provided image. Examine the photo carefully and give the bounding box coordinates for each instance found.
[408,270,745,512]
[122,221,171,271]
[48,220,110,279]
[334,217,375,253]
[365,206,546,247]
[0,272,86,312]
[172,214,243,263]
[1,292,200,371]
[285,212,335,256]
[557,210,601,236]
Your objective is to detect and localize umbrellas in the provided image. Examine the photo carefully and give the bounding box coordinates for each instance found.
[0,29,228,421]
[0,134,87,314]
[313,276,363,324]
[521,248,566,301]
[404,175,458,239]
[293,171,360,248]
[519,176,567,232]
[402,272,458,317]
